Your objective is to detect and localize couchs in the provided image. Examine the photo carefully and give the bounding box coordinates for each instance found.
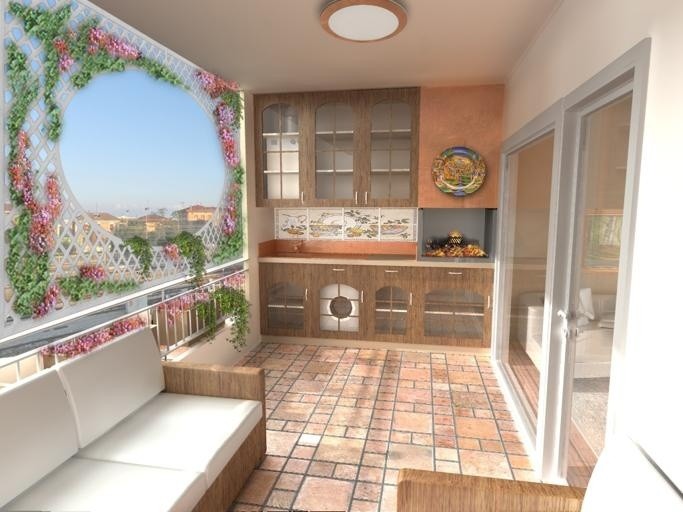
[0,322,266,511]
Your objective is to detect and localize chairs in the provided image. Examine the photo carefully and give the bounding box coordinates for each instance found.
[394,437,683,511]
[514,289,617,382]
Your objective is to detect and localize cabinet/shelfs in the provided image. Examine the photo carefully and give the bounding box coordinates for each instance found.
[251,92,308,208]
[258,263,313,338]
[365,266,418,344]
[308,86,421,209]
[313,265,366,340]
[417,267,494,348]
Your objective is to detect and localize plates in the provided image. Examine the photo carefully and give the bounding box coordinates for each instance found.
[429,147,488,197]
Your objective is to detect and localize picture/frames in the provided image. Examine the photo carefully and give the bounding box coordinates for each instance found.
[581,208,623,274]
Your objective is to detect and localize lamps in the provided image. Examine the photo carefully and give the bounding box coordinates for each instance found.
[319,0,408,44]
